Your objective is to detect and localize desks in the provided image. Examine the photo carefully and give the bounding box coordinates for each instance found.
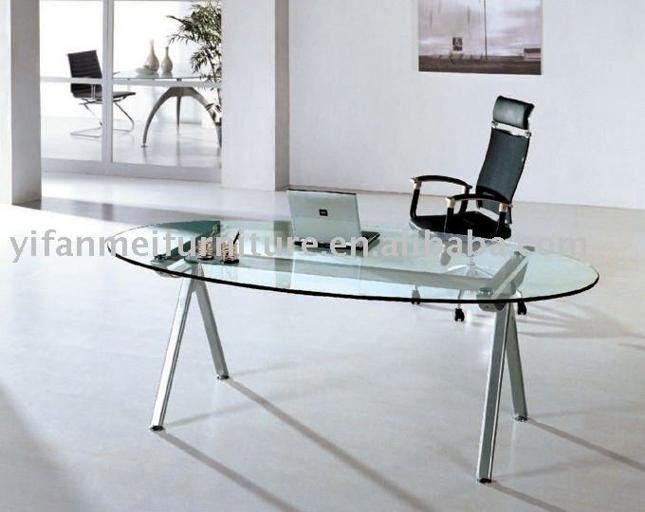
[105,220,601,484]
[112,70,223,147]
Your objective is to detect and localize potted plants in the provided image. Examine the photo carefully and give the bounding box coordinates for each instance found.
[164,0,223,146]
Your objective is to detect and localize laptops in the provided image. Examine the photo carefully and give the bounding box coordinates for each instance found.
[285,189,379,249]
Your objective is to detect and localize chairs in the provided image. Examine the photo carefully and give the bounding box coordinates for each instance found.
[67,51,135,138]
[407,96,534,321]
